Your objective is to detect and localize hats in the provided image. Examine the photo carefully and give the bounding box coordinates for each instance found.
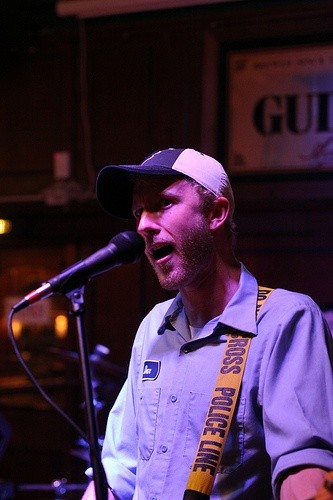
[95,147,236,230]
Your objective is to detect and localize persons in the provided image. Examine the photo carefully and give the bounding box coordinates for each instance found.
[76,146,332,500]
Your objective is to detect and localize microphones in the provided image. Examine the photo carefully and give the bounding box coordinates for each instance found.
[12,230,146,314]
